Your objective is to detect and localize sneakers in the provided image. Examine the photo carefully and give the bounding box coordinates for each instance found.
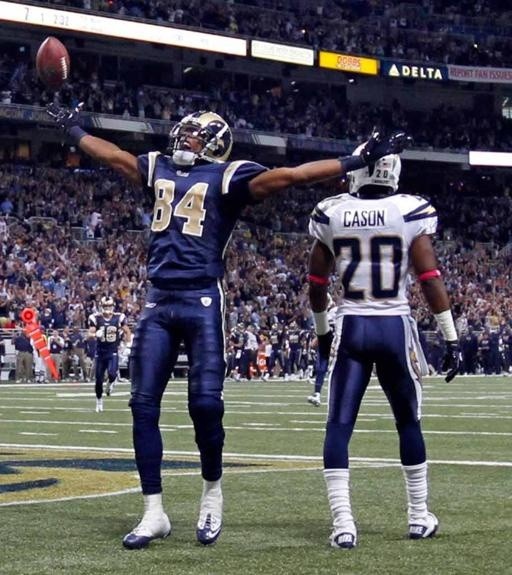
[196,490,224,546]
[327,527,358,550]
[122,511,172,551]
[95,398,104,413]
[408,511,440,540]
[307,392,321,407]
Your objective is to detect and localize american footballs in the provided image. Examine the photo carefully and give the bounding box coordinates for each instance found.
[37,35,69,85]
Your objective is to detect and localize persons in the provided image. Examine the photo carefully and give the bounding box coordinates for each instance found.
[44,95,410,547]
[409,188,512,378]
[220,183,339,381]
[0,163,155,412]
[307,139,463,550]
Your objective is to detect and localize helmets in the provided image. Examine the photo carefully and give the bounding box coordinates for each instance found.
[99,295,115,315]
[164,109,234,164]
[346,141,402,196]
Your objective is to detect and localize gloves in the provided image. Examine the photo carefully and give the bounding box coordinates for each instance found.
[440,339,465,384]
[316,328,335,361]
[45,99,86,138]
[360,121,408,166]
